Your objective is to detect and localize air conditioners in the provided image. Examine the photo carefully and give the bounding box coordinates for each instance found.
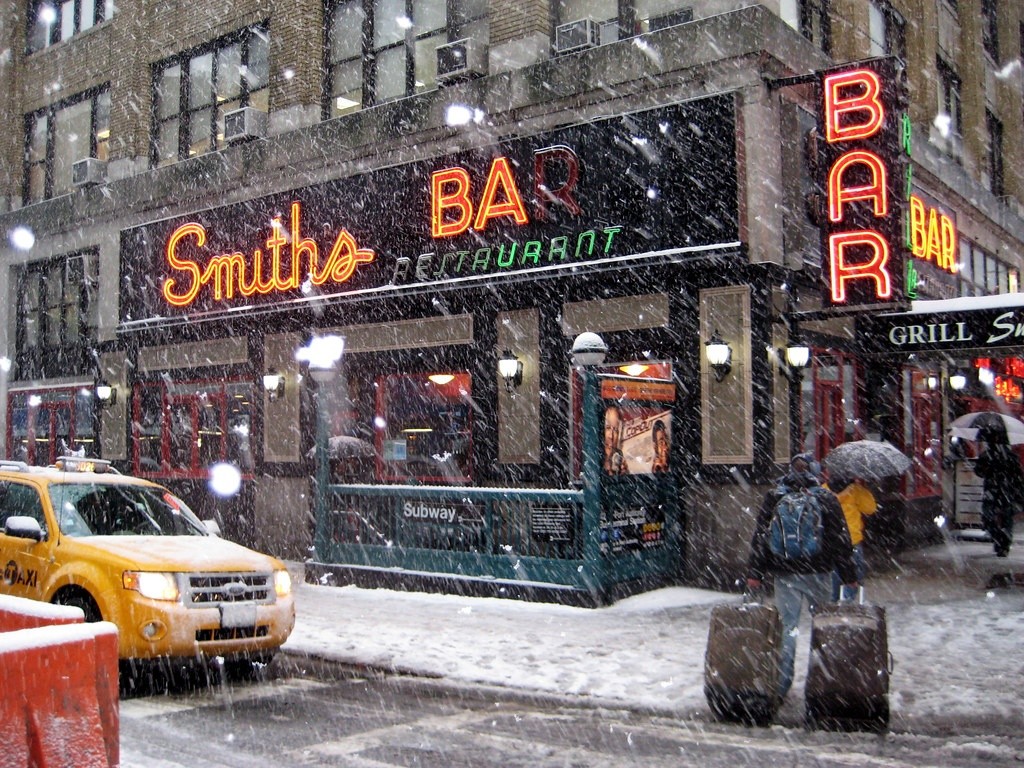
[435,38,489,83]
[72,157,107,187]
[555,19,601,53]
[65,255,97,284]
[224,107,268,145]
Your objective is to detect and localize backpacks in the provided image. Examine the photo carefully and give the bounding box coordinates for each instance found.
[767,483,828,562]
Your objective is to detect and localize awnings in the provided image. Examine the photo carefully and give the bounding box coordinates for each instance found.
[854,292,1024,362]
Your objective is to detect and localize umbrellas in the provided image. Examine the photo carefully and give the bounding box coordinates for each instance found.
[826,440,914,482]
[945,411,1024,445]
[305,435,375,458]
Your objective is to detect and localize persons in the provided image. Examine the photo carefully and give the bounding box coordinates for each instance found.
[652,420,671,473]
[973,442,1024,557]
[604,404,629,476]
[333,461,347,484]
[746,453,858,703]
[822,480,876,603]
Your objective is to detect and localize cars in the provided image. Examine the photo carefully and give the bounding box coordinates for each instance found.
[0,459,296,699]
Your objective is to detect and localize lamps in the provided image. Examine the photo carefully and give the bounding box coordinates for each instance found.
[263,363,286,402]
[703,330,732,383]
[96,378,116,408]
[498,349,523,394]
[572,332,609,372]
[948,365,966,389]
[778,329,811,385]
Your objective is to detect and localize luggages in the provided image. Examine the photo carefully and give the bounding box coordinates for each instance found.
[803,582,895,732]
[702,579,785,724]
